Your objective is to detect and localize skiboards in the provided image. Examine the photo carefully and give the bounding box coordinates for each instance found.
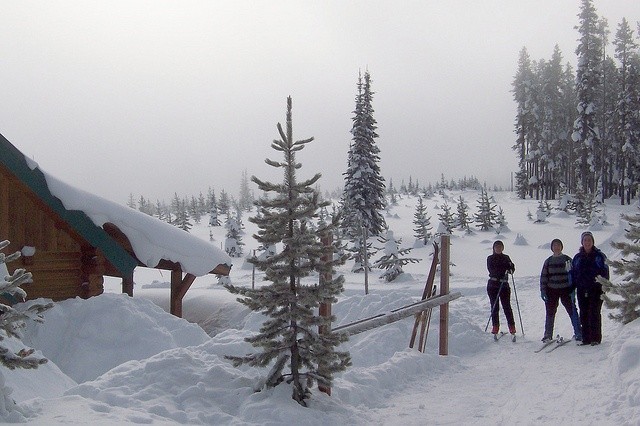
[493,331,516,343]
[533,334,571,354]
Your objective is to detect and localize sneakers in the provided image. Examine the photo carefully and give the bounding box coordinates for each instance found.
[508,324,516,334]
[491,324,499,334]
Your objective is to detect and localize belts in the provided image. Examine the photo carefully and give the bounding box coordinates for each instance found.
[490,277,507,282]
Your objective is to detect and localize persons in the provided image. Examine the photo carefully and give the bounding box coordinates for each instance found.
[485,240,516,335]
[568,231,609,346]
[540,239,584,342]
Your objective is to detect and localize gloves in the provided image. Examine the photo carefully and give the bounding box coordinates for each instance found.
[541,293,548,301]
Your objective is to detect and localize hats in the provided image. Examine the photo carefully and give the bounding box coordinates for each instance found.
[581,231,594,243]
